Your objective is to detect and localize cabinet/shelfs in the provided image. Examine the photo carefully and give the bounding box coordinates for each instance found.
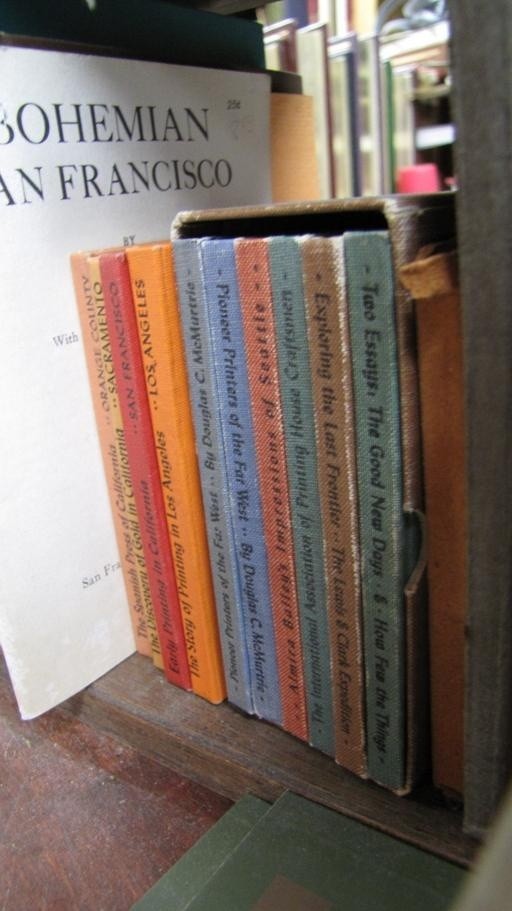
[4,0,512,871]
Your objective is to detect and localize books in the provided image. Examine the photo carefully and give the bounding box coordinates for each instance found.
[258,1,451,199]
[66,230,465,798]
